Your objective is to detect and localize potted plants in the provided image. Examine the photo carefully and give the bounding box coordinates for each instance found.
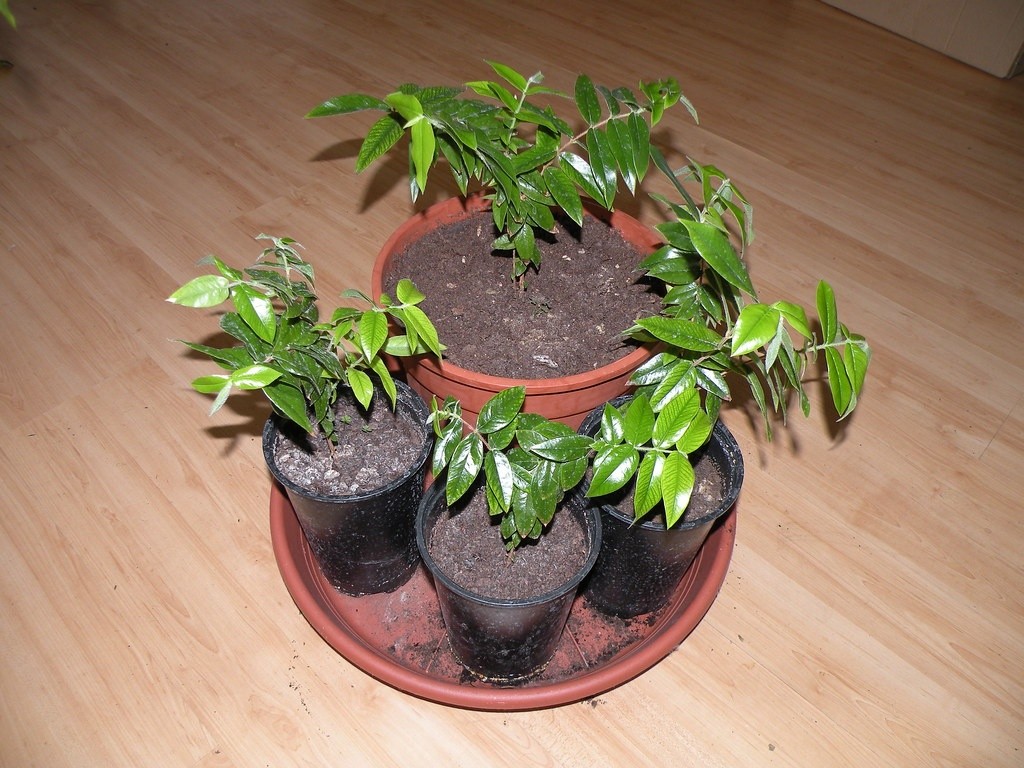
[302,59,700,451]
[414,387,711,684]
[163,234,450,596]
[574,164,871,617]
